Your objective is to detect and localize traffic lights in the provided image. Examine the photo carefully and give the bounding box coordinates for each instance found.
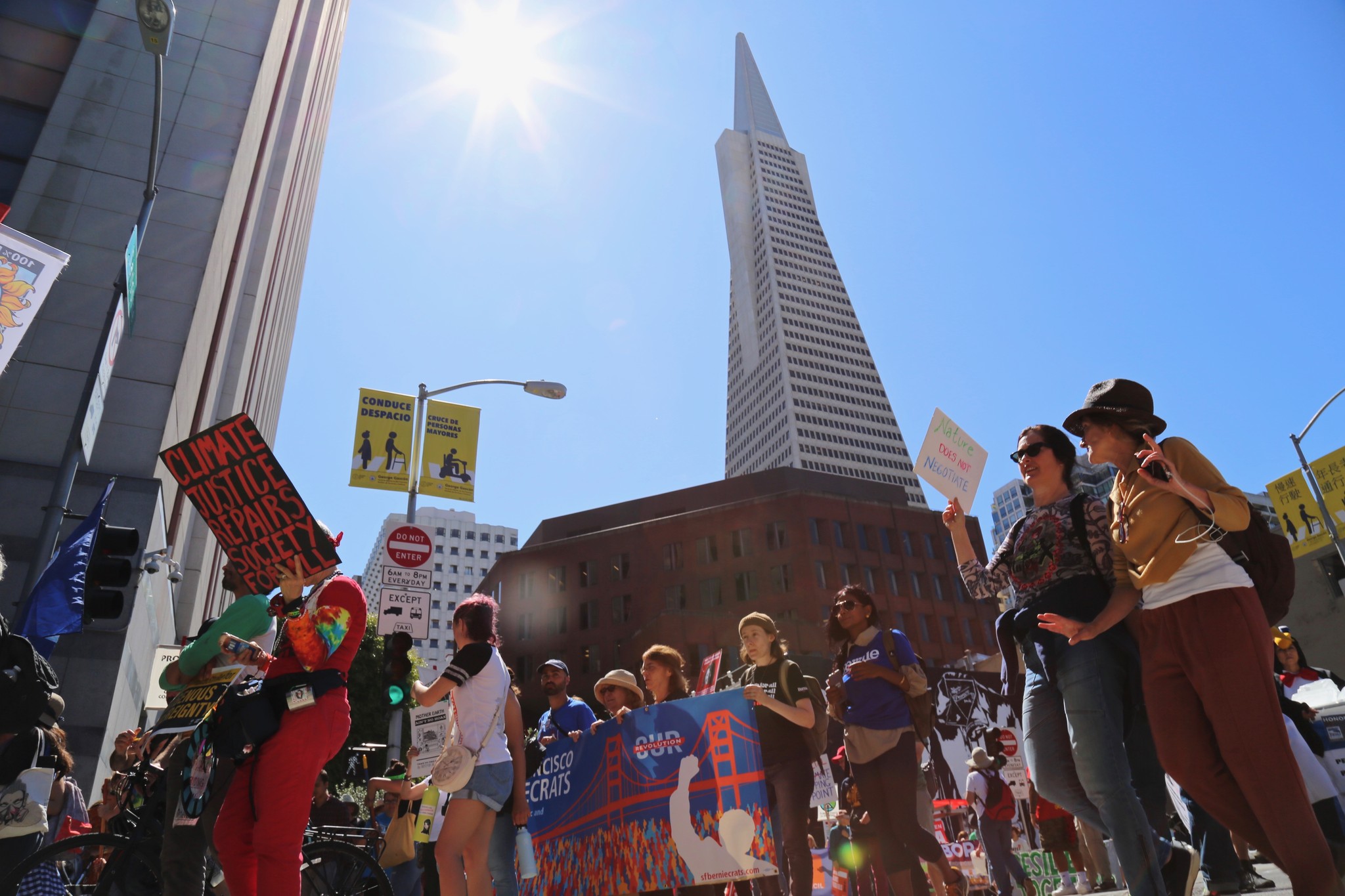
[984,726,1007,770]
[386,379,567,767]
[340,750,375,781]
[82,522,140,620]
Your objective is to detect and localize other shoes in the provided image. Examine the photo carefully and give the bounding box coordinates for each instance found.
[1075,876,1092,894]
[1094,879,1118,892]
[1051,879,1078,896]
[1239,859,1277,891]
[1204,891,1241,896]
[1160,845,1200,896]
[1024,877,1036,896]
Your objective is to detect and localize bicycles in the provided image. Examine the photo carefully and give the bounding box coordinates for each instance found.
[2,750,396,896]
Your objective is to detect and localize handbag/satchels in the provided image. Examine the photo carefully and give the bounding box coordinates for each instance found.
[211,668,348,766]
[0,727,55,839]
[431,746,476,793]
[377,795,416,869]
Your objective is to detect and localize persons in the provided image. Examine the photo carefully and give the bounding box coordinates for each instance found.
[362,579,546,896]
[0,519,368,896]
[822,378,1344,896]
[535,612,817,896]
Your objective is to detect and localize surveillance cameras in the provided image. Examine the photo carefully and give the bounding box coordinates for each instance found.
[166,568,183,583]
[145,559,160,574]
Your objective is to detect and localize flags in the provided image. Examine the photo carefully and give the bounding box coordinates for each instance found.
[20,477,115,662]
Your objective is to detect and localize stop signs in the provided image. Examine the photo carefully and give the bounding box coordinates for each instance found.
[383,520,436,572]
[996,727,1021,758]
[381,632,413,708]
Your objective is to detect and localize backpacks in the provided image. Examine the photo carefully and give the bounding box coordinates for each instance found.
[971,767,1016,821]
[833,628,936,742]
[0,616,59,733]
[522,719,547,780]
[1108,438,1295,628]
[744,659,829,763]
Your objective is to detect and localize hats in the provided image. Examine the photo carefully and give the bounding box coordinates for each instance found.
[965,746,994,768]
[339,793,359,816]
[537,659,569,676]
[38,692,65,730]
[375,799,383,812]
[831,745,845,764]
[1062,379,1167,438]
[594,669,644,706]
[738,611,777,639]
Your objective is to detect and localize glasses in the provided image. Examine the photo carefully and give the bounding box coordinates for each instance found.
[1116,502,1131,543]
[383,799,398,804]
[832,600,863,616]
[1010,442,1052,463]
[600,685,615,696]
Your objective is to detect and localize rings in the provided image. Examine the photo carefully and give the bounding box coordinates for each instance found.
[282,574,286,579]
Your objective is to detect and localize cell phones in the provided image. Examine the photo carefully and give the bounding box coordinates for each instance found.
[1135,457,1172,482]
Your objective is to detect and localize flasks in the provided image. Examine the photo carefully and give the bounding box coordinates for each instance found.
[413,778,440,843]
[516,824,536,879]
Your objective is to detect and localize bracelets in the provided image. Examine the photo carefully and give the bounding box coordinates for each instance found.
[282,596,304,615]
[898,676,907,686]
[366,795,375,803]
[402,773,412,781]
[286,611,301,617]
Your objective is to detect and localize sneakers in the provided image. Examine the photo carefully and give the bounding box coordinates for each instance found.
[942,866,969,896]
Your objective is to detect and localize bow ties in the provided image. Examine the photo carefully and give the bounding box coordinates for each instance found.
[1279,668,1319,688]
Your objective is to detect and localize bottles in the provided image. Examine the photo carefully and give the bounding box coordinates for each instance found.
[217,632,261,661]
